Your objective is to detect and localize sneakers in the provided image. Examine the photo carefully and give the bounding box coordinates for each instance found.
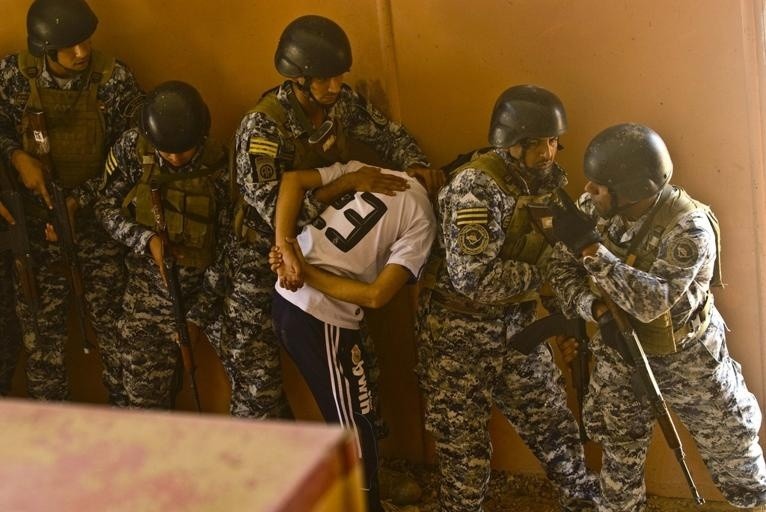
[377,465,422,511]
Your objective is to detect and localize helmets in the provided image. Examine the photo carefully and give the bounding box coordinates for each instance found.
[583,124,673,206]
[488,84,569,149]
[140,80,211,154]
[274,15,352,79]
[26,0,98,57]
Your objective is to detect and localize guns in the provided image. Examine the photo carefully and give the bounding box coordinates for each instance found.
[22,110,94,356]
[591,276,705,505]
[133,186,200,413]
[308,120,347,163]
[511,310,592,446]
[1,157,42,341]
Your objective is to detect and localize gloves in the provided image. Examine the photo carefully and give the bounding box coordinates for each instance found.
[551,206,603,260]
[599,308,640,369]
